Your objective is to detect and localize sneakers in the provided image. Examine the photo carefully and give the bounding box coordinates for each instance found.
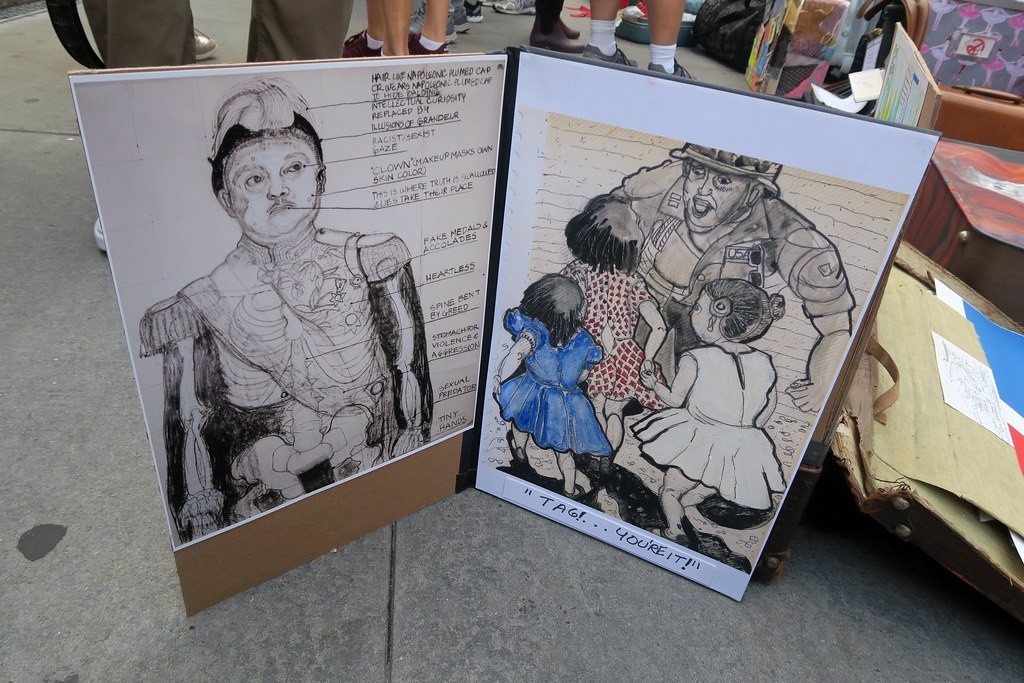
[583,44,638,69]
[647,58,696,81]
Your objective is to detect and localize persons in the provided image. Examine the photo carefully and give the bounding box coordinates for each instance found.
[82,0,536,250]
[530,0,585,54]
[583,0,697,81]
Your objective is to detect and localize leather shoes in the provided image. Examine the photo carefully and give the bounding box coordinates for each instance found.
[558,18,580,39]
[529,16,585,53]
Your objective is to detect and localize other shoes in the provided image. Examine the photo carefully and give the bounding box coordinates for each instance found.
[194,28,218,61]
[492,0,536,15]
[407,31,450,55]
[343,29,383,58]
[452,2,472,32]
[465,1,483,22]
[409,1,457,44]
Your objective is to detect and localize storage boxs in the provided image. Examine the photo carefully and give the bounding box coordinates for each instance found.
[614,13,697,47]
